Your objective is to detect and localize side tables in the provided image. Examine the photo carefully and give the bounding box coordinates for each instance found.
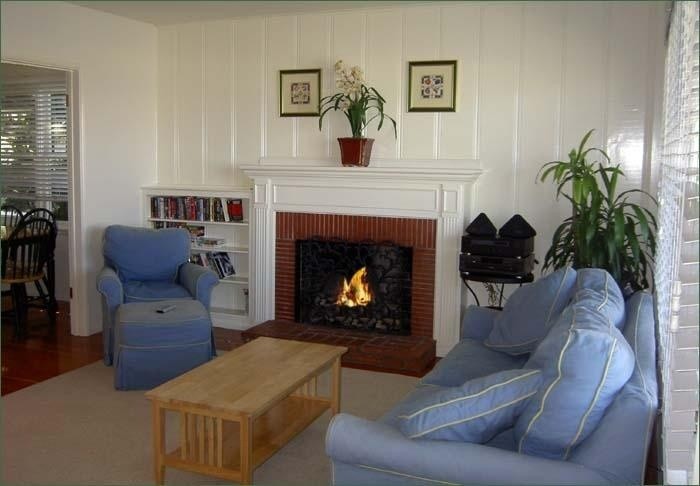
[457,271,534,311]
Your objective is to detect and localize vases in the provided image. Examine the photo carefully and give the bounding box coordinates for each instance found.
[338,137,374,168]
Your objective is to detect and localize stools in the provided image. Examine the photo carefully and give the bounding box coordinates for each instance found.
[113,300,212,391]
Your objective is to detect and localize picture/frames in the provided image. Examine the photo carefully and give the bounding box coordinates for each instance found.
[408,60,456,112]
[279,69,322,116]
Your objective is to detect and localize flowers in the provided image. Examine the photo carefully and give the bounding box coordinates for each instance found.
[316,60,399,138]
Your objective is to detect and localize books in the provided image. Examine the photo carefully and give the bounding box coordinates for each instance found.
[151,196,243,279]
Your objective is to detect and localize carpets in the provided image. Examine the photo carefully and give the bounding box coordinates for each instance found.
[0,351,424,486]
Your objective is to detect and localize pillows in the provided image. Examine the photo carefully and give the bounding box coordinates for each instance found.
[395,368,543,445]
[483,266,577,357]
[512,305,636,460]
[562,268,627,332]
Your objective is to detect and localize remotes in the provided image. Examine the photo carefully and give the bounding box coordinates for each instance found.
[156,304,177,313]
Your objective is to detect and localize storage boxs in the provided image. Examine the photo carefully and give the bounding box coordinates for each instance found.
[209,283,249,316]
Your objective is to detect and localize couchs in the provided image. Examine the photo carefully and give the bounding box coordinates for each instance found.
[324,267,660,486]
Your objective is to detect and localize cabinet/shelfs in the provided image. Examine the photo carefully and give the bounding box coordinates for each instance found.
[141,183,255,332]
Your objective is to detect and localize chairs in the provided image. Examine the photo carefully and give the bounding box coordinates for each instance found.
[96,225,219,366]
[0,205,59,341]
